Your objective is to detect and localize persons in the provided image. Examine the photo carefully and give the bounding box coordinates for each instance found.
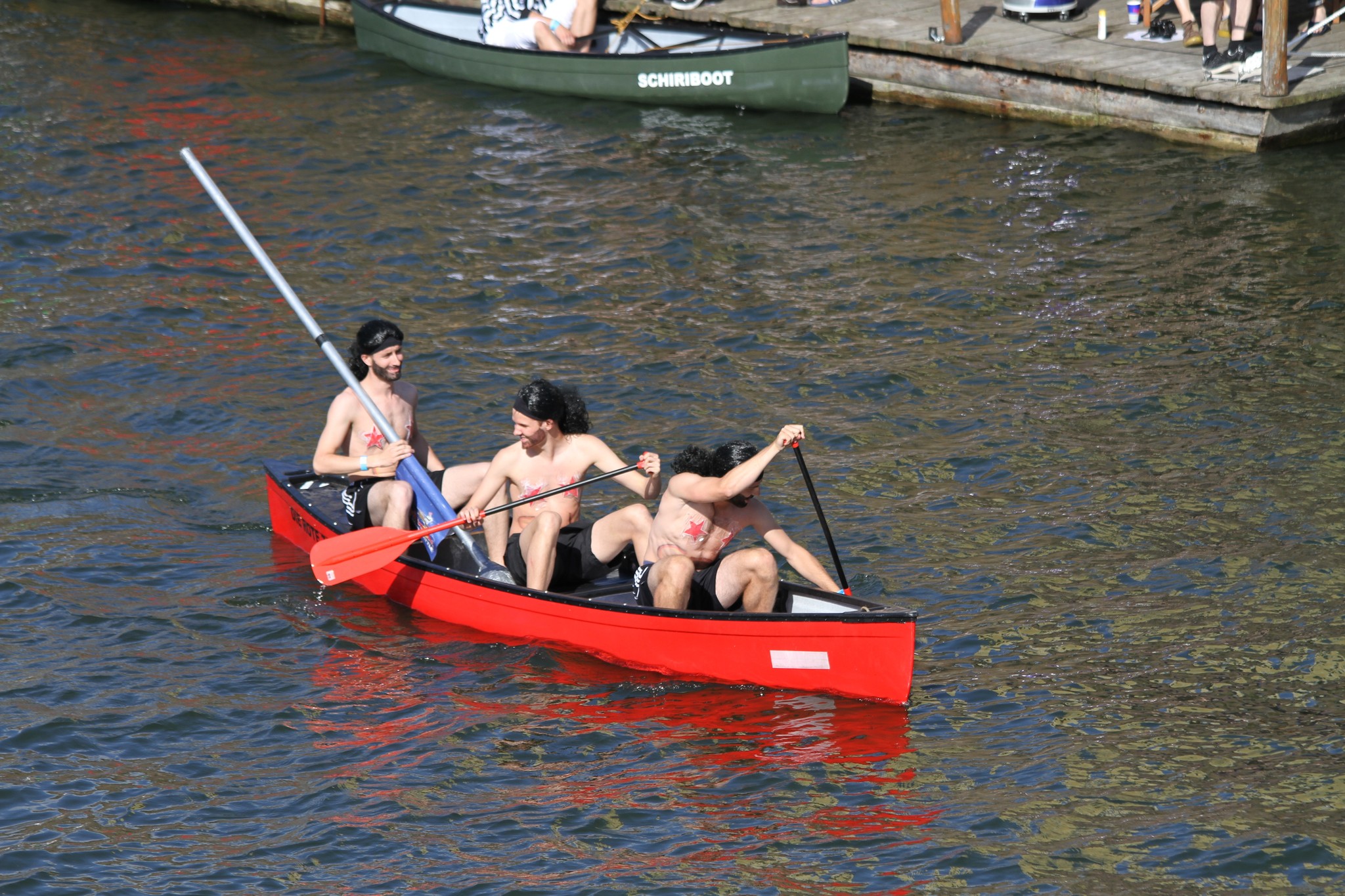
[1171,0,1331,69]
[311,319,510,569]
[475,0,597,54]
[456,378,662,597]
[632,424,846,613]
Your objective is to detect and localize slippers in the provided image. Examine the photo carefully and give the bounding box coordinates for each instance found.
[1254,18,1262,35]
[1300,18,1331,38]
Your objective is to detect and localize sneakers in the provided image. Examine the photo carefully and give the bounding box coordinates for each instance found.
[1200,49,1234,75]
[1224,42,1259,69]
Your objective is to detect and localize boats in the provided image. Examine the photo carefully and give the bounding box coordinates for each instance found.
[350,0,851,115]
[259,456,919,708]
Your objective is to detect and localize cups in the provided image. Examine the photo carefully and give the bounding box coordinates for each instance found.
[1126,0,1141,25]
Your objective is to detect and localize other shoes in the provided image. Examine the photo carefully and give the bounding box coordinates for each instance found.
[1217,16,1253,39]
[1181,20,1203,46]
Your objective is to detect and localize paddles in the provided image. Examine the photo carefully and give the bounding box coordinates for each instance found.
[783,439,852,596]
[309,451,654,586]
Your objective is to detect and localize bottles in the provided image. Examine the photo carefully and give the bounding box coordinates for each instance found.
[1097,9,1107,40]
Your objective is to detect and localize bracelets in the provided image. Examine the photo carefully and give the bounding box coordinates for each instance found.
[836,589,845,595]
[549,21,560,31]
[360,455,368,471]
